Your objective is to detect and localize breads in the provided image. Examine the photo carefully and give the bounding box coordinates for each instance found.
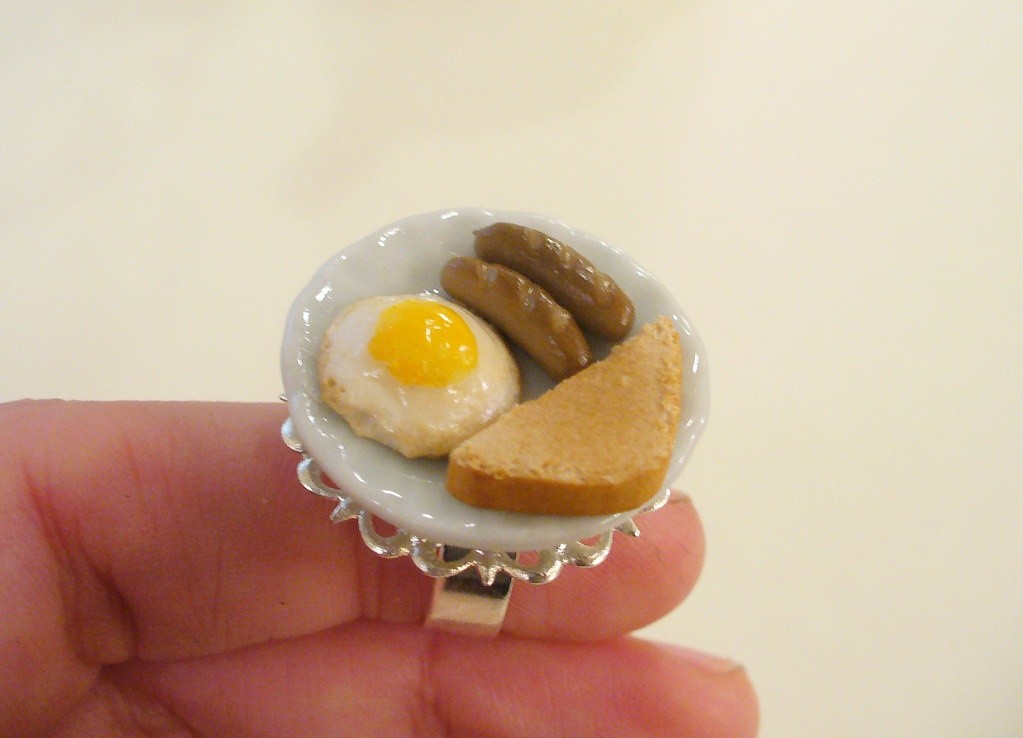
[446,316,683,515]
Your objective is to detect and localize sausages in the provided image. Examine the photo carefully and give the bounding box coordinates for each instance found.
[441,256,593,385]
[471,221,635,340]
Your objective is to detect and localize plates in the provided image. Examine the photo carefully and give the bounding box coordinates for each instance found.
[281,205,712,554]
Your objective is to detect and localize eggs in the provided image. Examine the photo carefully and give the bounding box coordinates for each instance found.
[317,292,523,459]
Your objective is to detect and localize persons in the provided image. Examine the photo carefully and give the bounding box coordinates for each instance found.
[1,398,758,738]
